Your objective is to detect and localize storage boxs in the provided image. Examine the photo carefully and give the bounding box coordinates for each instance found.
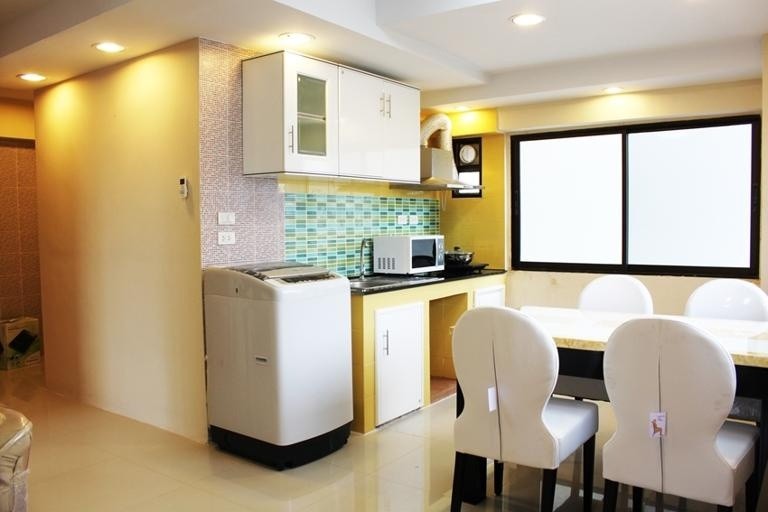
[1,318,42,371]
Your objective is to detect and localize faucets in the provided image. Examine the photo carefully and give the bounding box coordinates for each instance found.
[358,238,370,280]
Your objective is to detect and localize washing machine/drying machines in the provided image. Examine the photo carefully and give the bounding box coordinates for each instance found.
[203,261,354,471]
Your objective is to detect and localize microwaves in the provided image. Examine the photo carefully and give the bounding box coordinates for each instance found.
[373,234,445,275]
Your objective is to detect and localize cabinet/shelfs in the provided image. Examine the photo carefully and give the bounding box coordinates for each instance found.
[241,52,338,178]
[352,286,425,434]
[339,67,421,184]
[468,274,506,309]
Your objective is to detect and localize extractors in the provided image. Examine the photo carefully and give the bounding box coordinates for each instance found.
[388,147,485,192]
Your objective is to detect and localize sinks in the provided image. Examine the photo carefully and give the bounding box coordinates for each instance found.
[350,278,400,289]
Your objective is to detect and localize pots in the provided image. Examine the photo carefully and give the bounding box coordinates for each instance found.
[444,247,475,265]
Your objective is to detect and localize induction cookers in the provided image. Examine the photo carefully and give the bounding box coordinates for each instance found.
[446,264,488,275]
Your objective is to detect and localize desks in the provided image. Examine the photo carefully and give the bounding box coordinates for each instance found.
[519,306,766,430]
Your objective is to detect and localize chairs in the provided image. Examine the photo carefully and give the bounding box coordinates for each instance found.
[579,275,653,314]
[684,279,768,319]
[451,307,597,508]
[600,317,758,508]
